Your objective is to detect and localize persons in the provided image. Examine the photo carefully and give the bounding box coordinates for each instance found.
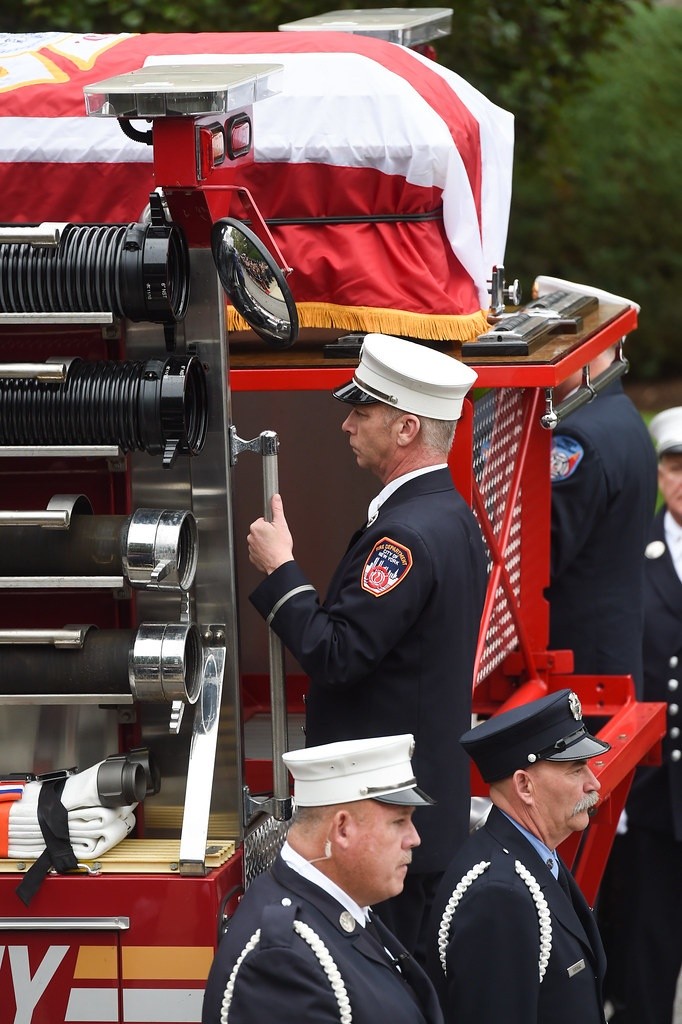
[200,733,446,1024]
[246,327,488,963]
[236,251,276,292]
[526,274,659,728]
[415,687,612,1024]
[615,400,682,1023]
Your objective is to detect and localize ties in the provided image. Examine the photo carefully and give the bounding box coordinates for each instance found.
[365,915,386,952]
[555,859,572,905]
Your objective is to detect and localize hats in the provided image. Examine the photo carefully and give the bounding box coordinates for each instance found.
[331,332,479,422]
[648,405,682,459]
[458,688,612,784]
[281,733,439,809]
[532,275,641,344]
